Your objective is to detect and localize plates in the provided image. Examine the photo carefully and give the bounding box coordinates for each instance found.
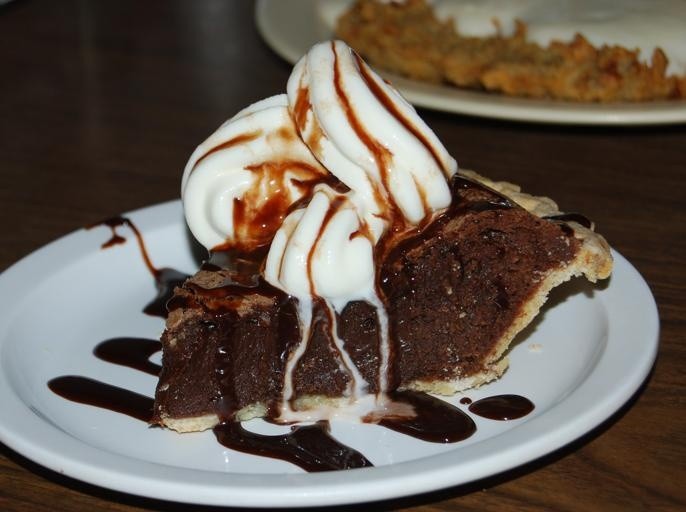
[255,2,685,127]
[1,196,661,510]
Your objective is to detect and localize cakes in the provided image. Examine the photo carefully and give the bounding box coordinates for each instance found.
[150,39,614,436]
